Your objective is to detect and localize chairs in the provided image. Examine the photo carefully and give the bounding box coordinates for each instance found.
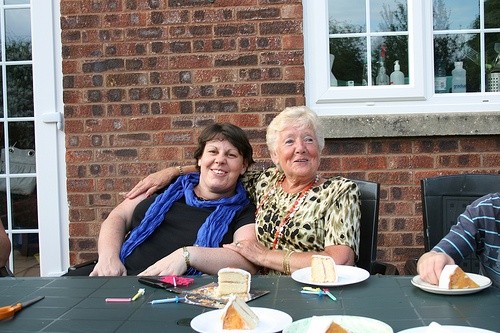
[403,172,500,276]
[353,180,401,275]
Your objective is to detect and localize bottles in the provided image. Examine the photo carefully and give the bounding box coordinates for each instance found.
[435,54,447,93]
[452,62,467,93]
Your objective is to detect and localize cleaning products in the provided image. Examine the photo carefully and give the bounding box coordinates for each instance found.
[435,57,467,93]
[375,59,405,85]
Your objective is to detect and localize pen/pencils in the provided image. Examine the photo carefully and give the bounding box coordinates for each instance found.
[301,286,337,300]
[152,296,185,305]
[132,288,145,301]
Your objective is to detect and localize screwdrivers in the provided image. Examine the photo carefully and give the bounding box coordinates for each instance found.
[0,295,45,322]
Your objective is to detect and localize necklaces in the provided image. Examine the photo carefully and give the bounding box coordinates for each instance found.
[254,168,321,252]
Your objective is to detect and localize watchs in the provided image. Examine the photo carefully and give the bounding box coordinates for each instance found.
[180,243,193,273]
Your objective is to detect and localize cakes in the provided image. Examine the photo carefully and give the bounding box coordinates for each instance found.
[439,264,481,289]
[324,321,350,333]
[220,296,259,330]
[218,267,251,294]
[311,254,337,283]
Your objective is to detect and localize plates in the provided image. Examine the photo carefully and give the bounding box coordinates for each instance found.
[411,272,493,295]
[282,314,394,333]
[395,324,499,333]
[191,307,293,333]
[290,265,371,287]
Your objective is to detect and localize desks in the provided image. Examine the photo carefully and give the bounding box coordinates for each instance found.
[0,275,500,333]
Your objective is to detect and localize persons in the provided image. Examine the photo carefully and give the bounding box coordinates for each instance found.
[414,189,500,288]
[0,218,15,278]
[123,104,363,280]
[58,123,263,280]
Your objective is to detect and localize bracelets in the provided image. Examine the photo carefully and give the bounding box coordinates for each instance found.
[177,165,184,176]
[282,248,295,275]
